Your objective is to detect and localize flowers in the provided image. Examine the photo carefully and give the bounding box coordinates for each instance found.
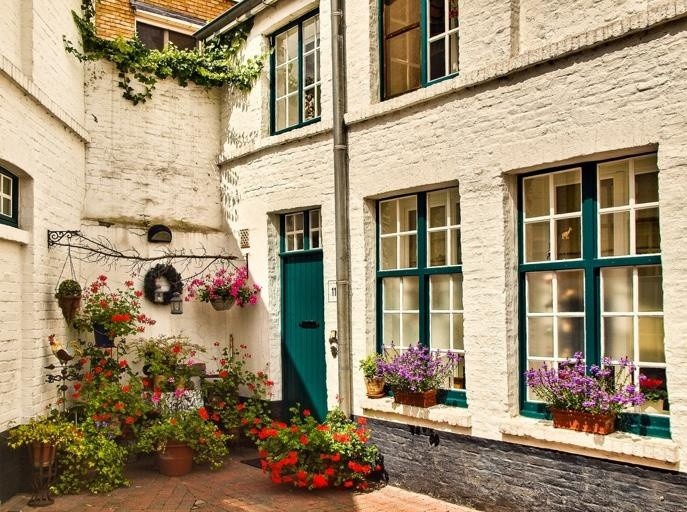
[523,352,647,416]
[76,275,156,339]
[42,333,275,440]
[373,342,462,394]
[257,402,384,492]
[184,265,262,308]
[128,406,234,473]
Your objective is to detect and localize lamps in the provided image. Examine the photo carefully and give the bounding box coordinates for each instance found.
[170,292,183,315]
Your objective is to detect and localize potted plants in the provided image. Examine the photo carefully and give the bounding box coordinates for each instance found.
[5,413,132,506]
[360,354,385,398]
[56,280,82,314]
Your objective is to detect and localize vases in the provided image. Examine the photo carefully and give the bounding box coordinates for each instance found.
[549,406,615,434]
[392,388,436,408]
[229,426,242,443]
[155,444,193,476]
[92,323,116,347]
[210,298,235,310]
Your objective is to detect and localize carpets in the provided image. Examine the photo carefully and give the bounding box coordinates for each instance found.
[240,458,270,471]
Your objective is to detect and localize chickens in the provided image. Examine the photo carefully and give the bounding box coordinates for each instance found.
[47,334,81,366]
